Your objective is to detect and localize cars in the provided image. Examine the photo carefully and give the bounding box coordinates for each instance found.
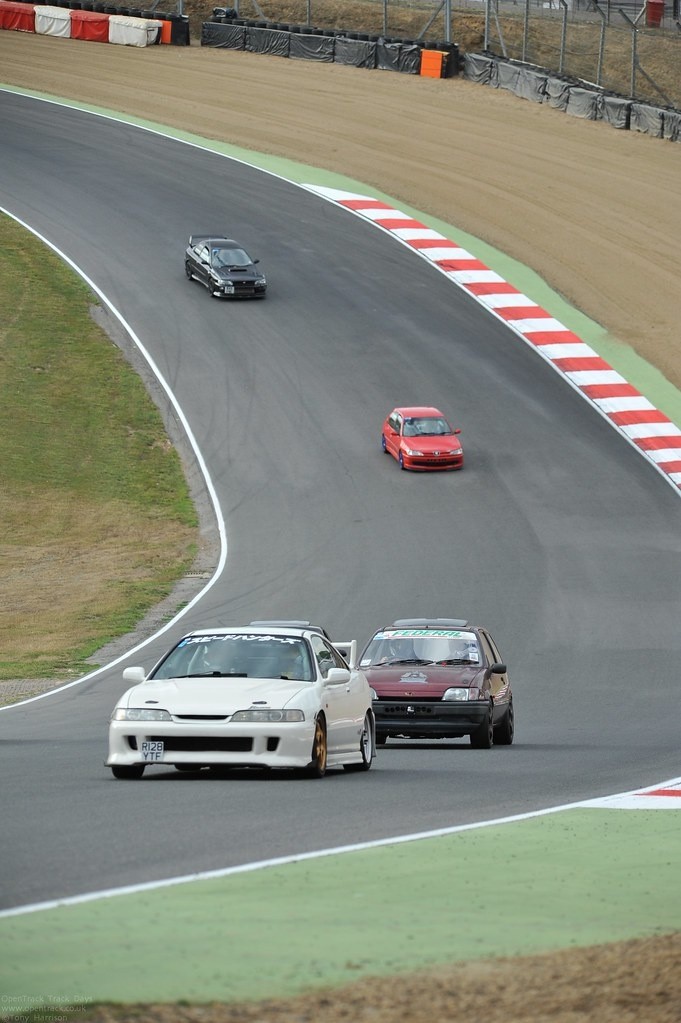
[185,234,267,299]
[358,620,515,749]
[382,406,465,472]
[105,618,379,779]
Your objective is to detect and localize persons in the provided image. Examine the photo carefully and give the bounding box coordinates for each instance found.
[381,640,408,662]
